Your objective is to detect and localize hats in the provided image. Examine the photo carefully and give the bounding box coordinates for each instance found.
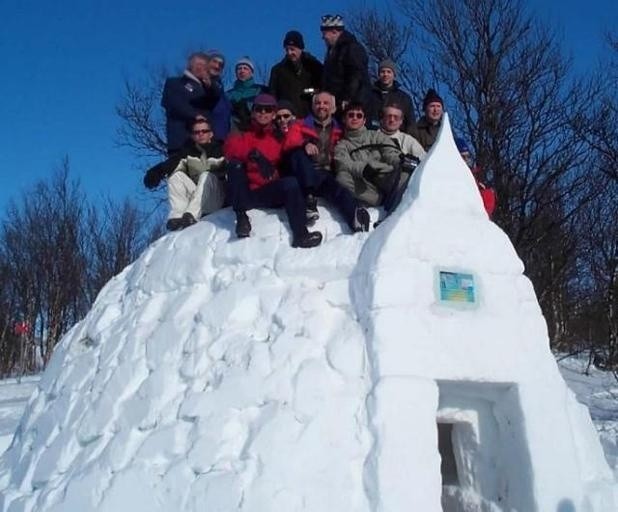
[423,88,444,110]
[203,49,255,77]
[378,56,397,77]
[284,31,304,49]
[320,14,345,31]
[455,138,469,152]
[252,93,297,115]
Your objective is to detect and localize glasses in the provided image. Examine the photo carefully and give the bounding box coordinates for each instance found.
[191,129,211,135]
[349,113,363,118]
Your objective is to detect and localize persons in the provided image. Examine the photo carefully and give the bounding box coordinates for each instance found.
[144,14,497,247]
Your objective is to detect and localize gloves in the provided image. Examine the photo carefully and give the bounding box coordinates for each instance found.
[363,163,383,186]
[144,168,161,189]
[398,152,420,163]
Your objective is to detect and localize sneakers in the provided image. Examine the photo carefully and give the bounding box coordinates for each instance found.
[236,209,251,237]
[292,195,322,248]
[350,205,370,231]
[166,212,197,231]
